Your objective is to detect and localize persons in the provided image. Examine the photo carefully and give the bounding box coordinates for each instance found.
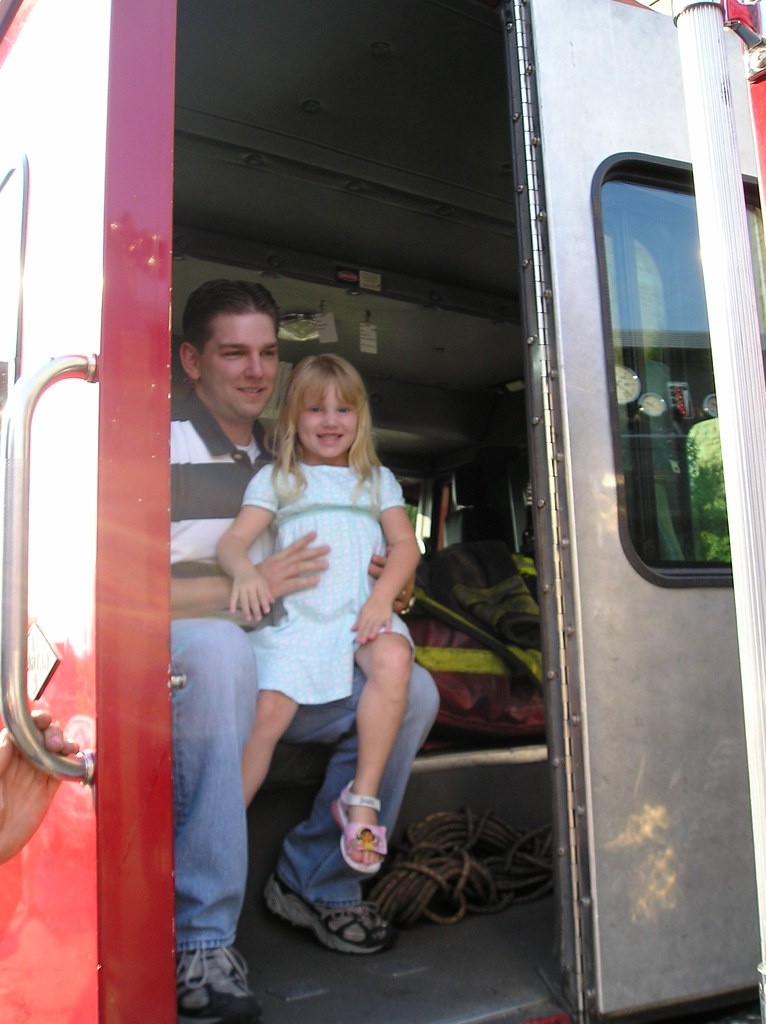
[168,280,440,1017]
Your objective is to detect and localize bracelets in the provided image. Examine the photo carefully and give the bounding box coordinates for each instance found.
[400,596,416,616]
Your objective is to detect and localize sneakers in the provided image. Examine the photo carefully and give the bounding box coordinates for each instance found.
[264,868,388,954]
[175,947,260,1024]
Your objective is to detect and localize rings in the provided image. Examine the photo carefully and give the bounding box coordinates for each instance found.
[398,591,406,600]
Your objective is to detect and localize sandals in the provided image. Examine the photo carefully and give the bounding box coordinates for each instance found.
[331,780,387,873]
